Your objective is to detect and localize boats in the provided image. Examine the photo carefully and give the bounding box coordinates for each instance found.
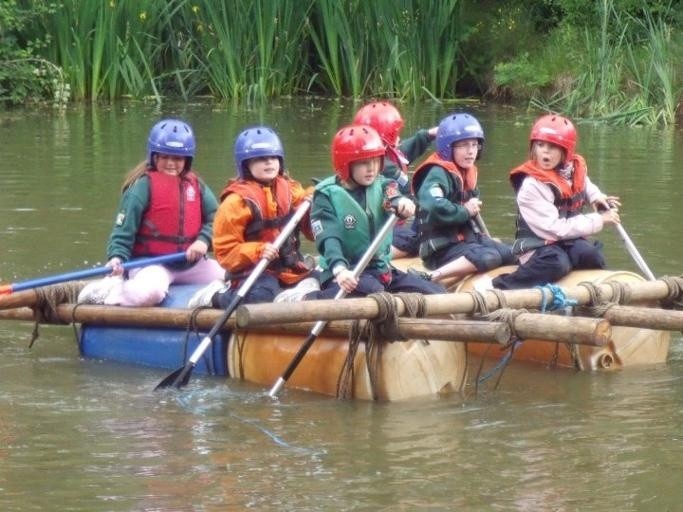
[0,255,683,405]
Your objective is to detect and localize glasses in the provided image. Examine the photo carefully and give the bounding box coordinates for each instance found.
[452,144,481,150]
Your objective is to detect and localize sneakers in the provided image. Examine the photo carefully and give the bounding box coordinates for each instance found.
[273,277,320,303]
[77,273,122,304]
[473,273,494,290]
[187,279,226,310]
[407,268,432,281]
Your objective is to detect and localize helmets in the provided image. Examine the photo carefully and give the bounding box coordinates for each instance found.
[330,124,386,181]
[233,126,286,179]
[352,102,405,147]
[435,112,484,161]
[146,118,196,171]
[529,115,577,169]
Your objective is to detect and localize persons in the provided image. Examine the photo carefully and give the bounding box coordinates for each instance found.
[410,113,514,289]
[351,101,439,260]
[272,124,447,304]
[77,119,226,307]
[472,114,622,291]
[187,126,315,310]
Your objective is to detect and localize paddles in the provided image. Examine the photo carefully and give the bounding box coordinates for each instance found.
[153,177,319,389]
